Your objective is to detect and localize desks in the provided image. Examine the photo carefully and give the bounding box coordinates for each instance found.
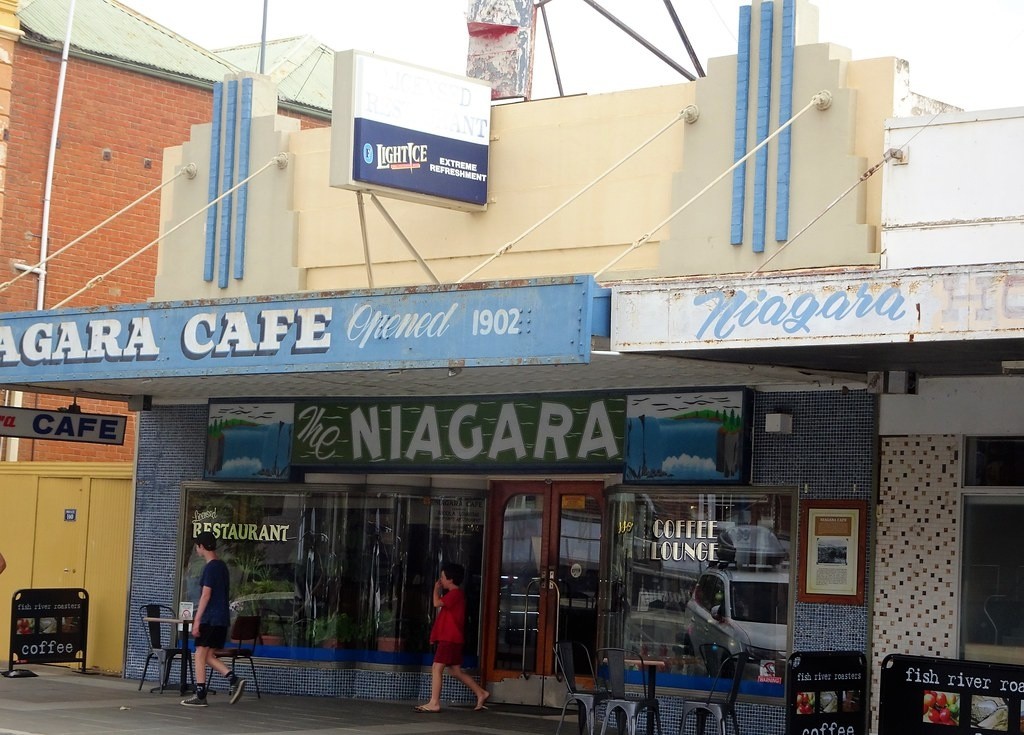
[142,617,217,696]
[602,658,700,735]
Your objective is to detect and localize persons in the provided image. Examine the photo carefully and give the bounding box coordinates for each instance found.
[412,564,490,713]
[181,532,247,707]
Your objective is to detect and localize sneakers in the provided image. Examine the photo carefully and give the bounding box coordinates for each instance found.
[229,677,247,705]
[180,694,208,707]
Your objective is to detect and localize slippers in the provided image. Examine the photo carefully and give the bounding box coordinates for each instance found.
[472,703,497,712]
[412,706,441,712]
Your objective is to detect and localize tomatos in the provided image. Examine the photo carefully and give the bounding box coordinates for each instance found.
[924,690,957,725]
[796,692,814,714]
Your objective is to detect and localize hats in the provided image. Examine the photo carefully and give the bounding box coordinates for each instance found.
[192,532,216,544]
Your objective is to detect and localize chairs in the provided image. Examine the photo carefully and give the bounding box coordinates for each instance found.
[137,604,195,694]
[595,648,661,735]
[678,652,749,735]
[207,616,261,699]
[552,640,613,735]
